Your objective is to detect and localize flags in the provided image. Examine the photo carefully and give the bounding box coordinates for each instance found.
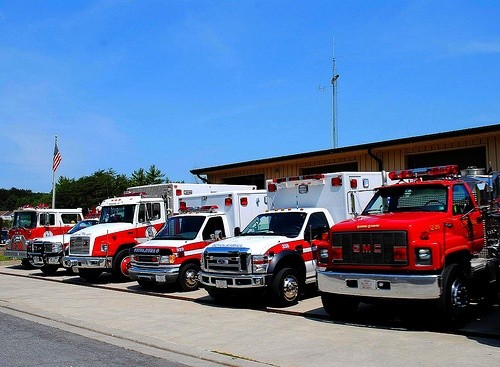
[52,136,63,173]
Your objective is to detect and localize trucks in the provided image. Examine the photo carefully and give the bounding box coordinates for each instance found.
[24,219,100,274]
[310,163,500,323]
[128,190,270,290]
[6,207,102,269]
[198,171,421,306]
[61,183,256,283]
